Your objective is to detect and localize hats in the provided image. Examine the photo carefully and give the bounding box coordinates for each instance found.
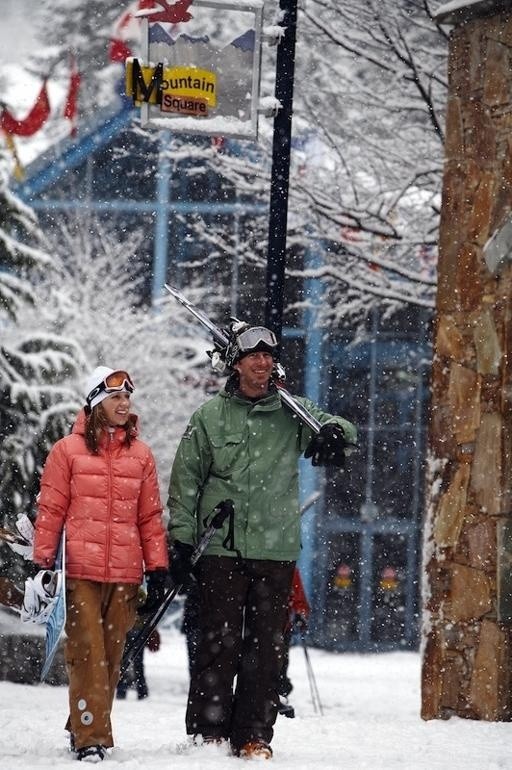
[85,365,132,412]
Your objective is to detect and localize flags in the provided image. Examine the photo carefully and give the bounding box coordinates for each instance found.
[0,0,154,140]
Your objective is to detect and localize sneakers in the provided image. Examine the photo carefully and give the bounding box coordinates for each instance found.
[193,732,229,747]
[77,743,108,763]
[231,738,273,762]
[69,731,80,755]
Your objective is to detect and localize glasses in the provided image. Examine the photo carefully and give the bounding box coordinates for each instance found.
[102,369,136,391]
[236,325,279,353]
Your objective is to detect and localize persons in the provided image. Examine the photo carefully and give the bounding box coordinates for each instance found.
[114,587,154,699]
[34,366,172,761]
[165,316,360,761]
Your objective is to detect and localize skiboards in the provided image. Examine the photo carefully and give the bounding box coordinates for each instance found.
[165,284,359,455]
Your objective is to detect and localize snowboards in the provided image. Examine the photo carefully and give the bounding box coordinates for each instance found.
[40,525,68,681]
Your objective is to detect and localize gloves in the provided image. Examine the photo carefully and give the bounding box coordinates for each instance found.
[143,570,168,608]
[304,423,346,468]
[169,542,197,586]
